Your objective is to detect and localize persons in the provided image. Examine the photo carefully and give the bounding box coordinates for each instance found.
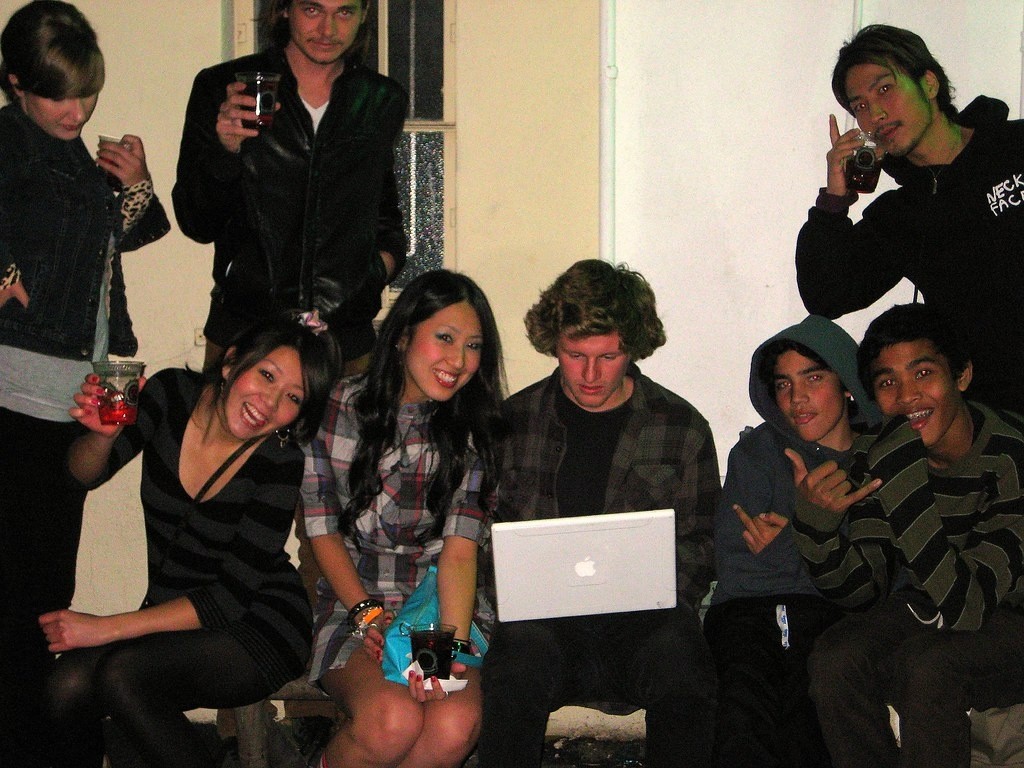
[0,0,1024,768]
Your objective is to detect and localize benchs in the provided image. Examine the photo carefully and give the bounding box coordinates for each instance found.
[232,671,646,767]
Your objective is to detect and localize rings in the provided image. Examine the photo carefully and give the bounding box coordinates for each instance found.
[371,623,379,629]
[444,691,449,700]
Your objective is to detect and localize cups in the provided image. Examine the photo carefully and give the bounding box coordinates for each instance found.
[99,134,132,191]
[91,360,143,425]
[406,623,457,681]
[234,69,283,131]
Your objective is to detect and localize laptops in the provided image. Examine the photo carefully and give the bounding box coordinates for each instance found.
[491,508,679,623]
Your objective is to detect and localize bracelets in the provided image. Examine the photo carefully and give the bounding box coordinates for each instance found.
[347,598,382,627]
[356,606,383,635]
[453,642,470,655]
